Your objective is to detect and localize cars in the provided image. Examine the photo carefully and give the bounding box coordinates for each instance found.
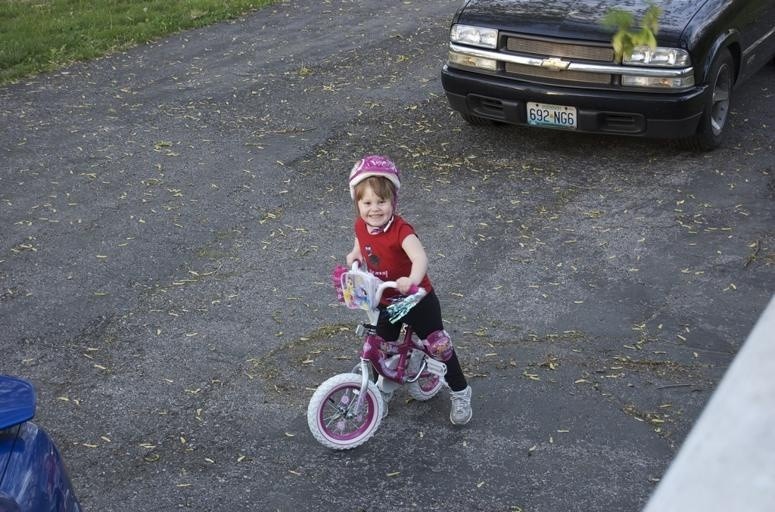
[0,375,81,512]
[441,1,775,152]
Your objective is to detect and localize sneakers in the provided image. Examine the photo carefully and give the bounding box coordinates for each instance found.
[450,385,472,425]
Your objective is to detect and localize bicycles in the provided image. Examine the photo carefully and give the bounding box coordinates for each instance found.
[308,258,449,451]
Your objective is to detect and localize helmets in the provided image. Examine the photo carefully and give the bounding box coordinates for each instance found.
[348,156,401,198]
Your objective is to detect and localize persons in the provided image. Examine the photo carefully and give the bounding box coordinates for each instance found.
[345,155,473,426]
